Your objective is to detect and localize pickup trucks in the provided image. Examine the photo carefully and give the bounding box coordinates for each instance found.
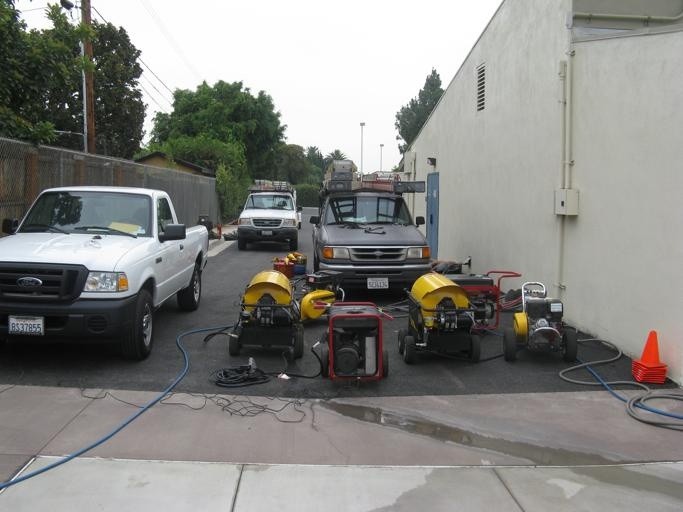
[0,185,209,362]
[309,190,431,297]
[237,192,302,251]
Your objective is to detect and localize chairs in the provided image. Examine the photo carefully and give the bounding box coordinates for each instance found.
[132,209,148,229]
[278,201,287,208]
[256,202,264,208]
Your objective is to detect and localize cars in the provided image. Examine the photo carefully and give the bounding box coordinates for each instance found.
[376,172,401,184]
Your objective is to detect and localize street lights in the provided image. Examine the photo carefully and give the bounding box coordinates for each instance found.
[380,144,384,170]
[360,122,366,179]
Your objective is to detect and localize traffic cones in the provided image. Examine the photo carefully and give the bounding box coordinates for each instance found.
[631,330,666,385]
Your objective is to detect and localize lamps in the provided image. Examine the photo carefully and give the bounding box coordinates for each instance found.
[427,158,436,166]
